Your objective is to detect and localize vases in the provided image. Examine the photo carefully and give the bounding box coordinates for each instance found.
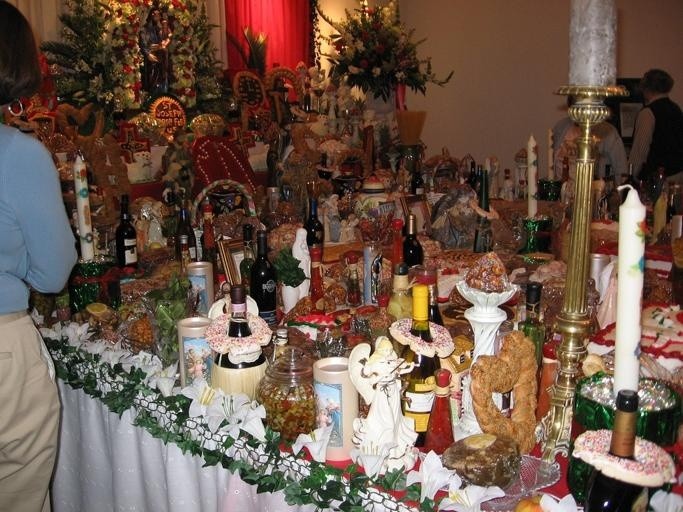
[364,87,396,116]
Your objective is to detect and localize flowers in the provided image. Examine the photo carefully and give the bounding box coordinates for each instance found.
[311,1,455,100]
[41,0,225,111]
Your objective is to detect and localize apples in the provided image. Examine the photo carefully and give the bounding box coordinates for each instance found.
[149,242,162,249]
[515,495,542,512]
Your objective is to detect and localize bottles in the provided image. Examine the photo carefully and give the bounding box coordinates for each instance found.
[239,223,255,297]
[411,159,425,197]
[467,160,494,253]
[254,328,318,445]
[249,229,278,328]
[114,194,139,270]
[210,284,271,369]
[517,281,545,385]
[302,197,325,261]
[386,213,455,454]
[620,163,675,234]
[583,388,650,512]
[175,198,218,285]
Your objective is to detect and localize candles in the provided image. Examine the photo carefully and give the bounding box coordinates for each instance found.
[73,153,95,263]
[615,183,646,400]
[548,130,555,181]
[569,0,617,86]
[527,135,537,219]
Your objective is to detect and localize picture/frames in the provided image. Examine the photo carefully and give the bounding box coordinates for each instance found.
[219,237,256,287]
[400,195,432,236]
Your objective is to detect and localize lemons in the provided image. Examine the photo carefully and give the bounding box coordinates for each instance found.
[86,300,107,316]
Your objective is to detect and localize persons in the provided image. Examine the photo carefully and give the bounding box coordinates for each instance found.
[626,68,682,179]
[1,1,79,511]
[551,93,628,186]
